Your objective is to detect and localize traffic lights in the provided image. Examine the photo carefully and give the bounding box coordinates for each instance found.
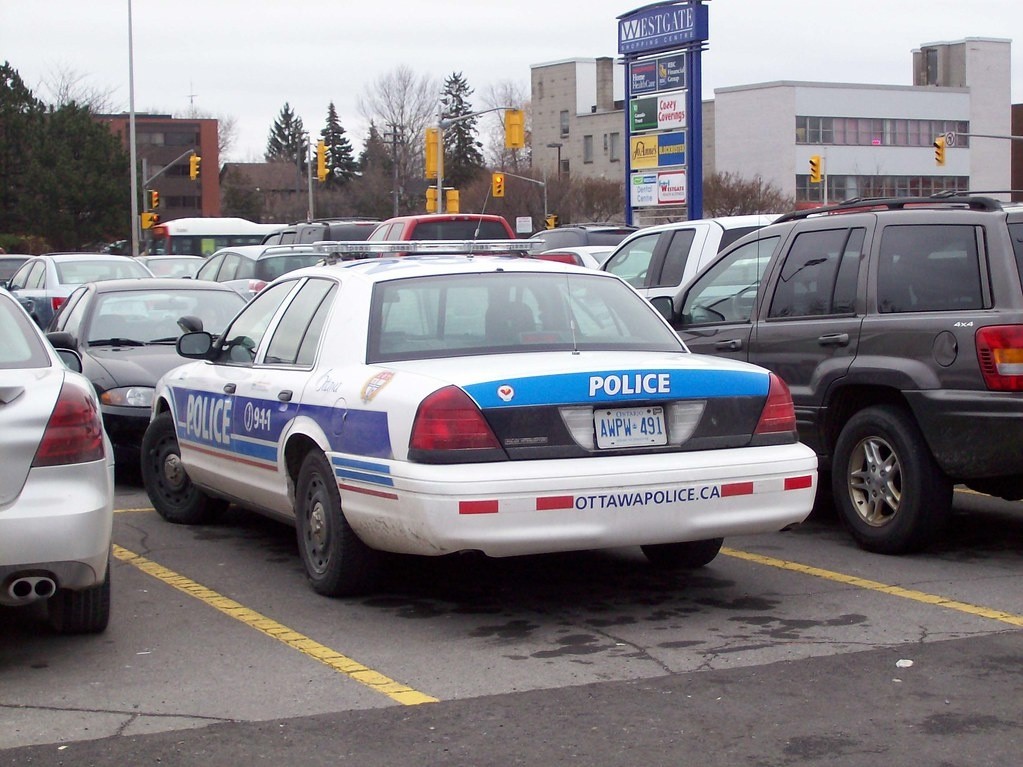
[492,173,504,198]
[190,154,201,182]
[544,214,558,230]
[933,138,945,166]
[152,191,160,210]
[809,156,822,183]
[140,212,160,230]
[317,140,329,183]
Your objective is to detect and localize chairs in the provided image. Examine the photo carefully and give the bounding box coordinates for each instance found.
[486,302,537,342]
[796,250,972,317]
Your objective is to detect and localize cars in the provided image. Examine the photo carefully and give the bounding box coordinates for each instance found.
[645,194,1023,554]
[0,286,115,639]
[40,279,252,485]
[139,242,819,597]
[0,196,1023,636]
